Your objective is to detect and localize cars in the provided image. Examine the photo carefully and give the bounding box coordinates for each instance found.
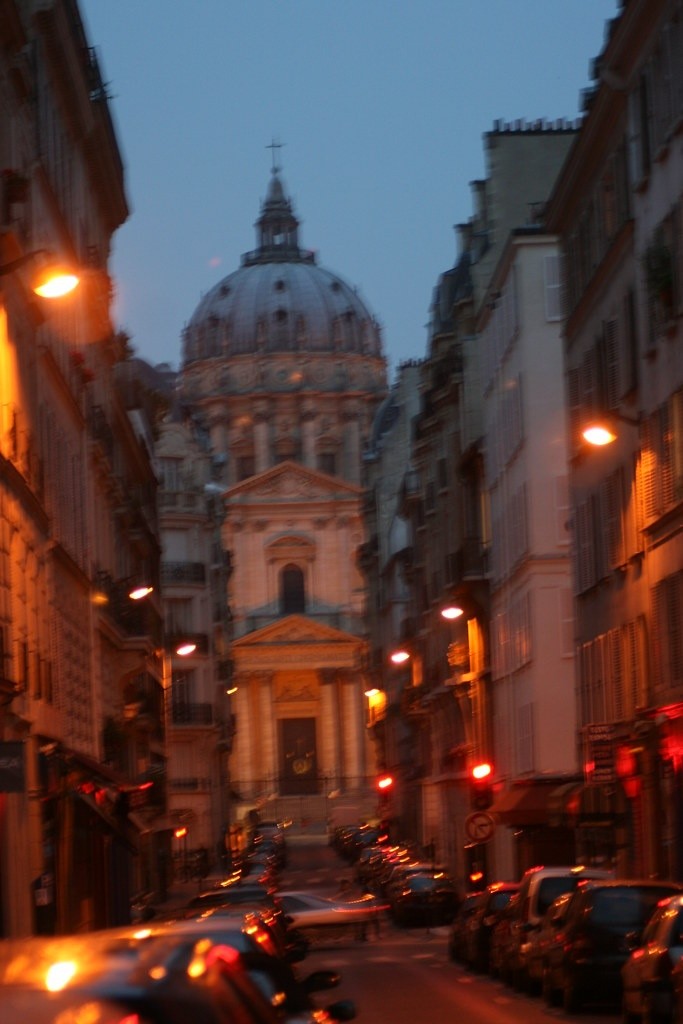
[0,812,357,1024]
[330,818,683,1024]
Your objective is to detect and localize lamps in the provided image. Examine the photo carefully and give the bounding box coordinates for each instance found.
[111,572,153,601]
[0,246,81,300]
[582,408,640,447]
